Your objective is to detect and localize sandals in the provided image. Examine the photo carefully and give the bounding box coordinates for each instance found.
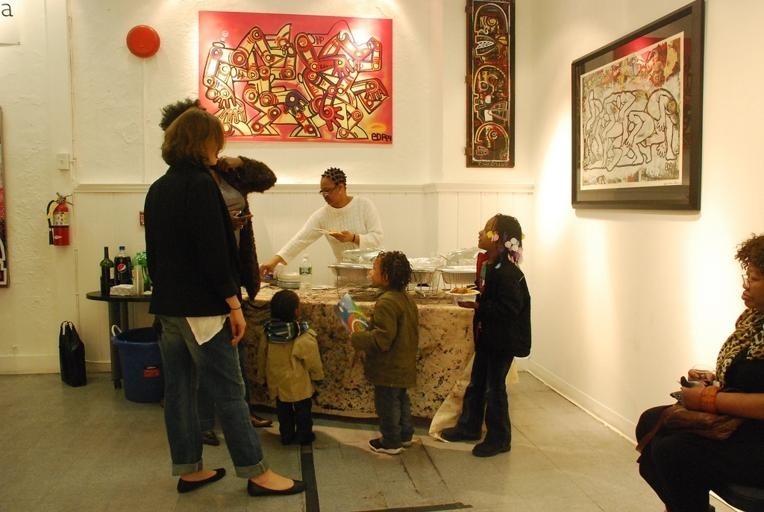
[177,467,226,494]
[247,477,308,498]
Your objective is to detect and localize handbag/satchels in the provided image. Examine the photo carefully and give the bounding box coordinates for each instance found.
[636,402,743,454]
[58,320,87,388]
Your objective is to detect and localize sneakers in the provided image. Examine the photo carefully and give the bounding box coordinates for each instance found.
[440,422,482,443]
[368,437,403,455]
[383,432,413,448]
[281,429,295,446]
[294,431,316,445]
[472,440,511,458]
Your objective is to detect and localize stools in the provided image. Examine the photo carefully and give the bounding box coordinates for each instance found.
[714,483,763,512]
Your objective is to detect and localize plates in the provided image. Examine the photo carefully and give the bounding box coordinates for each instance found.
[276,272,300,289]
[445,290,479,296]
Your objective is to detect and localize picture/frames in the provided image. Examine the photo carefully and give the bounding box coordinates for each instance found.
[566,0,707,216]
[464,0,519,171]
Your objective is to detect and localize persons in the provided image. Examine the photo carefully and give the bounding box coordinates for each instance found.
[439,214,531,456]
[158,98,271,445]
[143,109,308,495]
[259,165,382,275]
[347,250,418,454]
[635,234,763,511]
[259,289,323,445]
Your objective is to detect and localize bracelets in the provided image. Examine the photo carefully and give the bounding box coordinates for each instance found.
[700,386,719,413]
[230,305,242,312]
[352,233,355,243]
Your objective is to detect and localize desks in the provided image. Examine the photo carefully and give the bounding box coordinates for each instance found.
[84,291,161,392]
[229,279,482,429]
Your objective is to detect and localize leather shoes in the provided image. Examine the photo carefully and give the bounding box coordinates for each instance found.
[194,422,220,446]
[241,410,273,427]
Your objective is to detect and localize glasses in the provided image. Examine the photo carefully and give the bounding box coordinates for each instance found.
[320,186,337,195]
[742,273,761,289]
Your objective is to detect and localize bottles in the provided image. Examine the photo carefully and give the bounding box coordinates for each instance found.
[299,254,311,301]
[97,245,150,295]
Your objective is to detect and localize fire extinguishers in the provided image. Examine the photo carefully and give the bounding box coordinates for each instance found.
[48,192,74,245]
